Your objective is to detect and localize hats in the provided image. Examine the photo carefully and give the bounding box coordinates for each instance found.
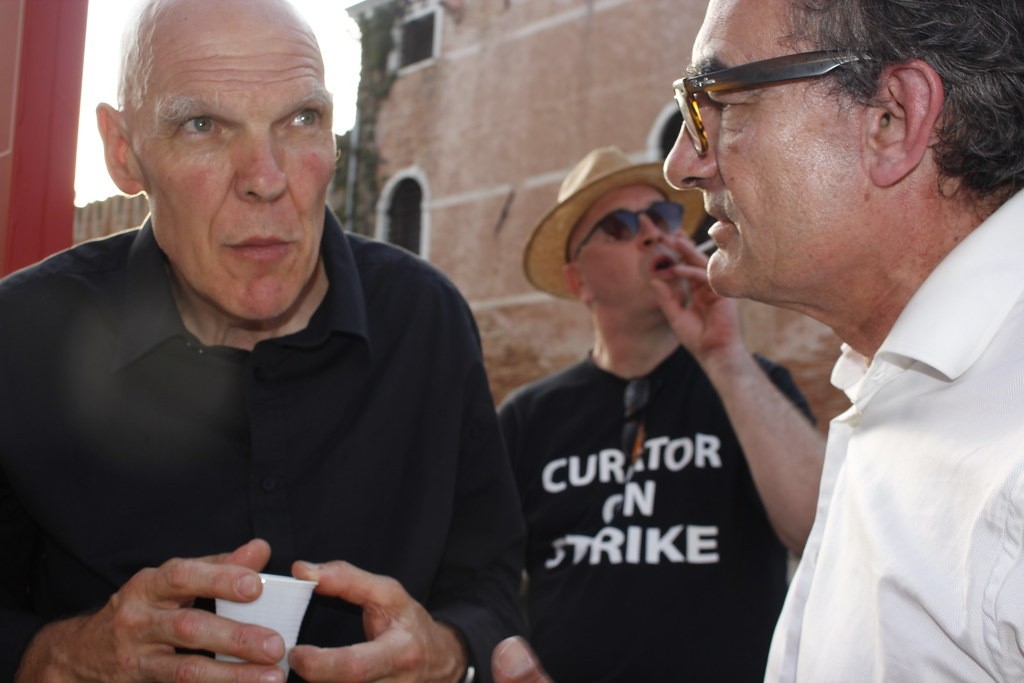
[523,147,705,299]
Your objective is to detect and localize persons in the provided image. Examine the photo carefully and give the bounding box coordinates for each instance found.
[457,146,826,683]
[0,0,525,683]
[663,0,1024,681]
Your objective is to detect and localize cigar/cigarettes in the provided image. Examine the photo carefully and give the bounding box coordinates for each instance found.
[676,239,715,264]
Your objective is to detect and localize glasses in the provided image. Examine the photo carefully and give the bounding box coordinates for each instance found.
[572,201,685,262]
[670,52,876,155]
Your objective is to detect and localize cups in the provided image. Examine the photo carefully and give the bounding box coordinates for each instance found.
[215,573,318,681]
